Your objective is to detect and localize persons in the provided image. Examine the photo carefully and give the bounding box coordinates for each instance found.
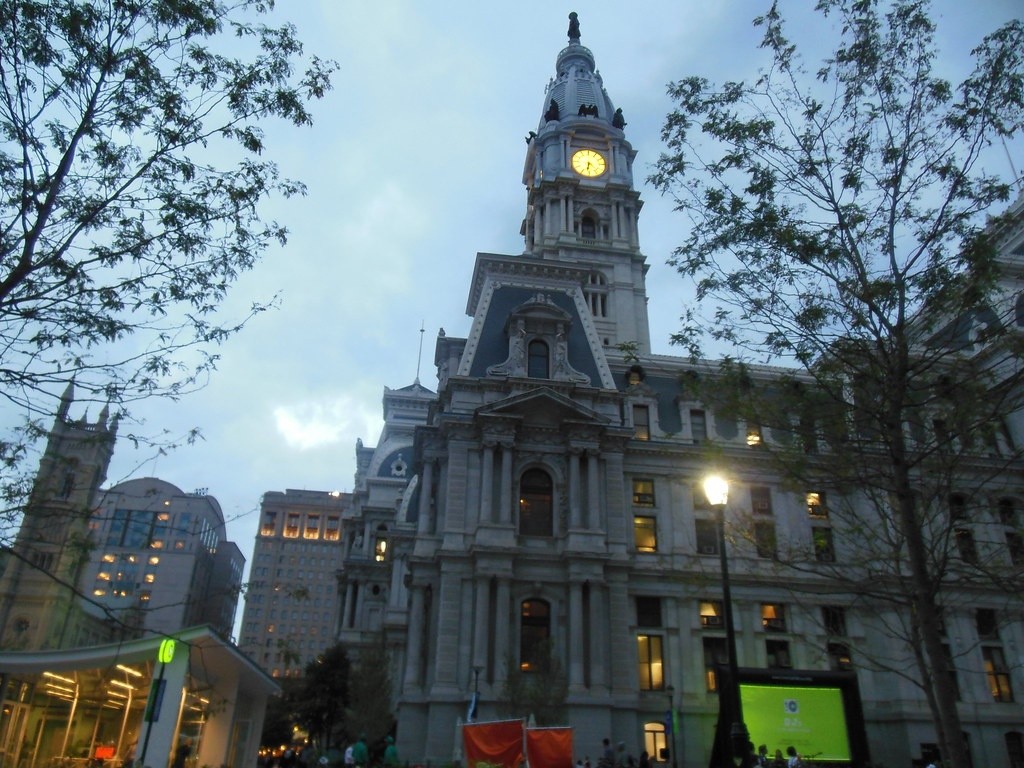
[258,732,405,768]
[573,737,653,767]
[922,752,944,767]
[171,736,194,767]
[740,740,803,768]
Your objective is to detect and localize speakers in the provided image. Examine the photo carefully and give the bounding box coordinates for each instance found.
[660,748,670,759]
[920,744,938,764]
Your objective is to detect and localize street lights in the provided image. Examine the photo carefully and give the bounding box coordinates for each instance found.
[693,464,752,767]
[666,684,678,768]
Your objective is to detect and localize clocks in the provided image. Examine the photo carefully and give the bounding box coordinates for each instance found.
[572,149,609,181]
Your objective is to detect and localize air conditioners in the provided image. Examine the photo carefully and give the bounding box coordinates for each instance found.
[637,495,649,502]
[706,616,720,625]
[757,502,769,513]
[812,505,824,515]
[768,619,781,628]
[702,546,715,555]
[820,553,832,562]
[781,659,792,667]
[715,655,729,665]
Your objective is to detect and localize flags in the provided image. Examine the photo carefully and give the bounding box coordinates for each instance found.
[527,726,574,768]
[459,718,525,768]
